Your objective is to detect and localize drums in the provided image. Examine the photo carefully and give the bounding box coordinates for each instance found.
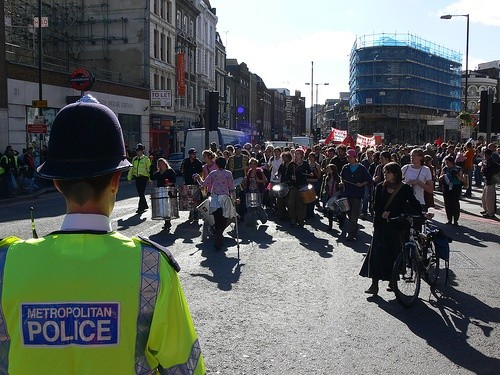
[150,186,179,220]
[326,196,338,211]
[272,183,289,197]
[179,184,201,211]
[196,196,216,226]
[246,193,264,208]
[235,177,246,193]
[335,197,351,211]
[299,185,318,203]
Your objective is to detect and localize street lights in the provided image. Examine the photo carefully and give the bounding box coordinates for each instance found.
[440,13,470,142]
[305,81,330,127]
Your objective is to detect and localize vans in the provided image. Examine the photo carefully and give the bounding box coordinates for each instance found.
[184,127,248,160]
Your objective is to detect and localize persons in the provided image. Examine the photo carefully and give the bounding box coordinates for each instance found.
[0,140,51,199]
[0,94,206,375]
[127,136,500,295]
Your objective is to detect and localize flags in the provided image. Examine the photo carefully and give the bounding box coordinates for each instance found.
[323,130,334,145]
[343,135,354,147]
[437,138,441,146]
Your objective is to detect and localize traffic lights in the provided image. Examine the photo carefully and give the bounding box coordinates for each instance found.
[208,91,220,132]
[477,90,488,133]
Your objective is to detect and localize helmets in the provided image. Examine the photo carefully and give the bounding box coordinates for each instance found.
[37,94,134,181]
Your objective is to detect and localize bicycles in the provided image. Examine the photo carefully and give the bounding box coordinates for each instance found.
[383,210,440,308]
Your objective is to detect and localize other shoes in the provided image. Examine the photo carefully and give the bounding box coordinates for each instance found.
[483,214,494,218]
[480,211,486,214]
[162,222,171,229]
[387,283,398,291]
[136,206,148,213]
[446,220,451,225]
[266,207,322,228]
[348,231,355,242]
[193,221,200,228]
[262,214,267,224]
[462,193,471,197]
[454,221,458,226]
[215,239,222,250]
[365,286,378,294]
[189,215,193,222]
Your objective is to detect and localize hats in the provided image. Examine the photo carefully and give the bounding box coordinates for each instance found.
[189,148,196,153]
[136,145,143,150]
[345,149,357,157]
